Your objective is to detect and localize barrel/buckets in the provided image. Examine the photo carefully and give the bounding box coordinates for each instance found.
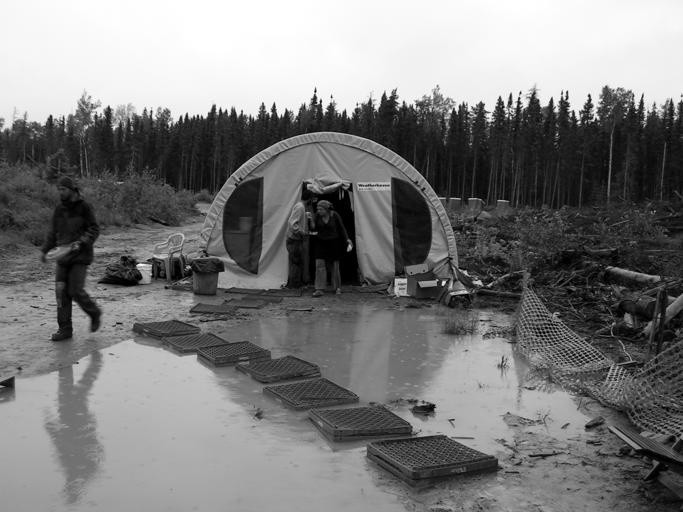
[136,263,153,285]
[238,216,252,232]
[192,268,219,296]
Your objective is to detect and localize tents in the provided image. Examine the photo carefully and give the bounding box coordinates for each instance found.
[188,127,465,299]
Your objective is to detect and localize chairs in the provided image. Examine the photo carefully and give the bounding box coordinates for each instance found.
[149,233,185,281]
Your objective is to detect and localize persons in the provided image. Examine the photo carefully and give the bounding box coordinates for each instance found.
[37,173,105,343]
[285,190,318,291]
[303,200,355,298]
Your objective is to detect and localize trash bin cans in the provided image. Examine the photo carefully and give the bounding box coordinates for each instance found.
[497,200,510,210]
[468,198,482,210]
[192,259,219,296]
[438,198,447,207]
[449,198,461,209]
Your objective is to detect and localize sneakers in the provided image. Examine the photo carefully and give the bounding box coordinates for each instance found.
[91,309,101,332]
[312,290,325,297]
[52,328,72,341]
[336,288,342,295]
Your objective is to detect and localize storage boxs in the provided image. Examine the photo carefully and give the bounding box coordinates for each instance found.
[404,264,440,299]
